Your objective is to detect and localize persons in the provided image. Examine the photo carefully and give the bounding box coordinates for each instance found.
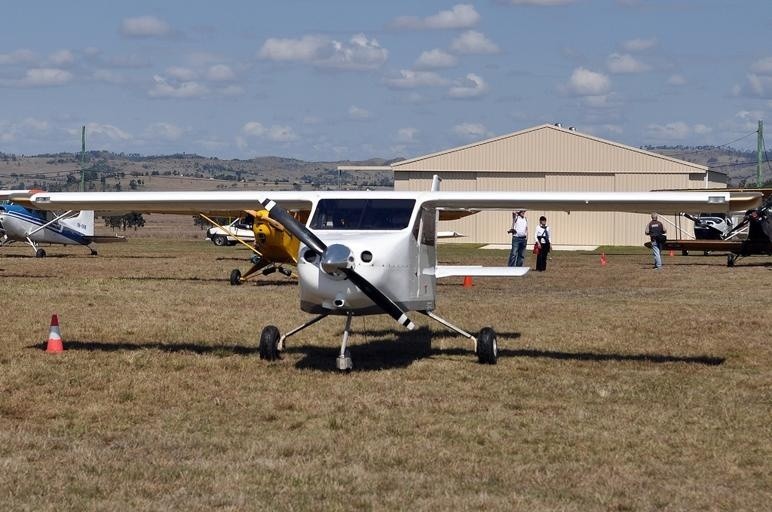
[508,211,528,267]
[534,216,552,271]
[645,212,667,269]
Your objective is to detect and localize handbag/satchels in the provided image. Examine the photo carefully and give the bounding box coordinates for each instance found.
[533,242,540,254]
[508,229,516,234]
[655,234,666,243]
